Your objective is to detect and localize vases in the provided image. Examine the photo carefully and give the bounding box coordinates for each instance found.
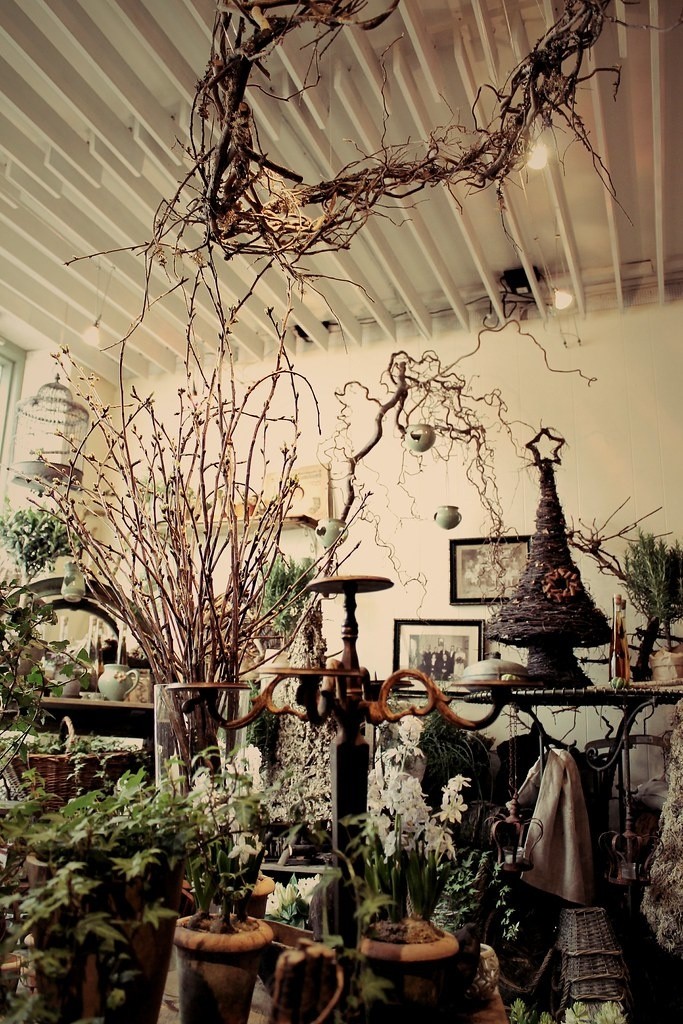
[153,682,251,799]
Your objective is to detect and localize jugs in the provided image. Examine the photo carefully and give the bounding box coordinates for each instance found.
[97,664,139,701]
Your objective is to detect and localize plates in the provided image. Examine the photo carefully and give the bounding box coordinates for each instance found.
[452,679,543,693]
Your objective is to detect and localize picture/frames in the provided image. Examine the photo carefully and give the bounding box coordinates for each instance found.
[390,619,486,700]
[449,535,532,606]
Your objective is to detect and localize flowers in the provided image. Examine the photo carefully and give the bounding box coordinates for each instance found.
[358,713,472,927]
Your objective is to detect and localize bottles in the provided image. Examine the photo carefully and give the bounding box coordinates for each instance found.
[59,616,68,655]
[609,594,629,683]
[98,620,104,691]
[80,616,97,690]
[60,562,85,602]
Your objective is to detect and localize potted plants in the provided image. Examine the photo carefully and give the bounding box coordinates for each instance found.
[255,550,313,665]
[429,844,521,1013]
[622,526,683,682]
[0,733,313,1024]
[358,930,460,1023]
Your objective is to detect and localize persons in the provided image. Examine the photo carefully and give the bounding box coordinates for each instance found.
[418,645,466,681]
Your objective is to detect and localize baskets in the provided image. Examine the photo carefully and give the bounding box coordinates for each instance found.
[0,716,131,824]
[550,905,635,1024]
